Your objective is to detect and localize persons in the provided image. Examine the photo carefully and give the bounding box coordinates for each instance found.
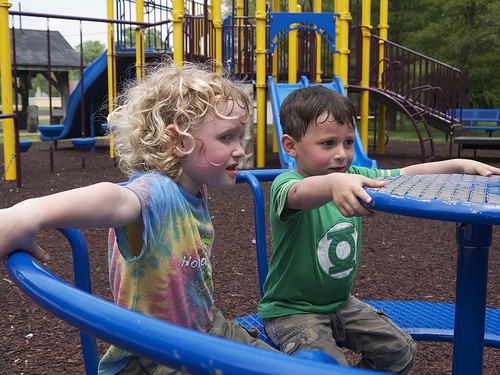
[0,54,277,375]
[258,84,500,375]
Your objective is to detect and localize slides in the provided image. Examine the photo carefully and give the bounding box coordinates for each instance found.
[267,65,378,175]
[37,41,126,142]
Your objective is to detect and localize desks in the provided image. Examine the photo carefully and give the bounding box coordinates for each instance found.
[358,173,500,375]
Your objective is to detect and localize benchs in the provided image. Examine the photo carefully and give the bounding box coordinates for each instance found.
[4,169,500,375]
[445,109,500,142]
[454,136,500,158]
